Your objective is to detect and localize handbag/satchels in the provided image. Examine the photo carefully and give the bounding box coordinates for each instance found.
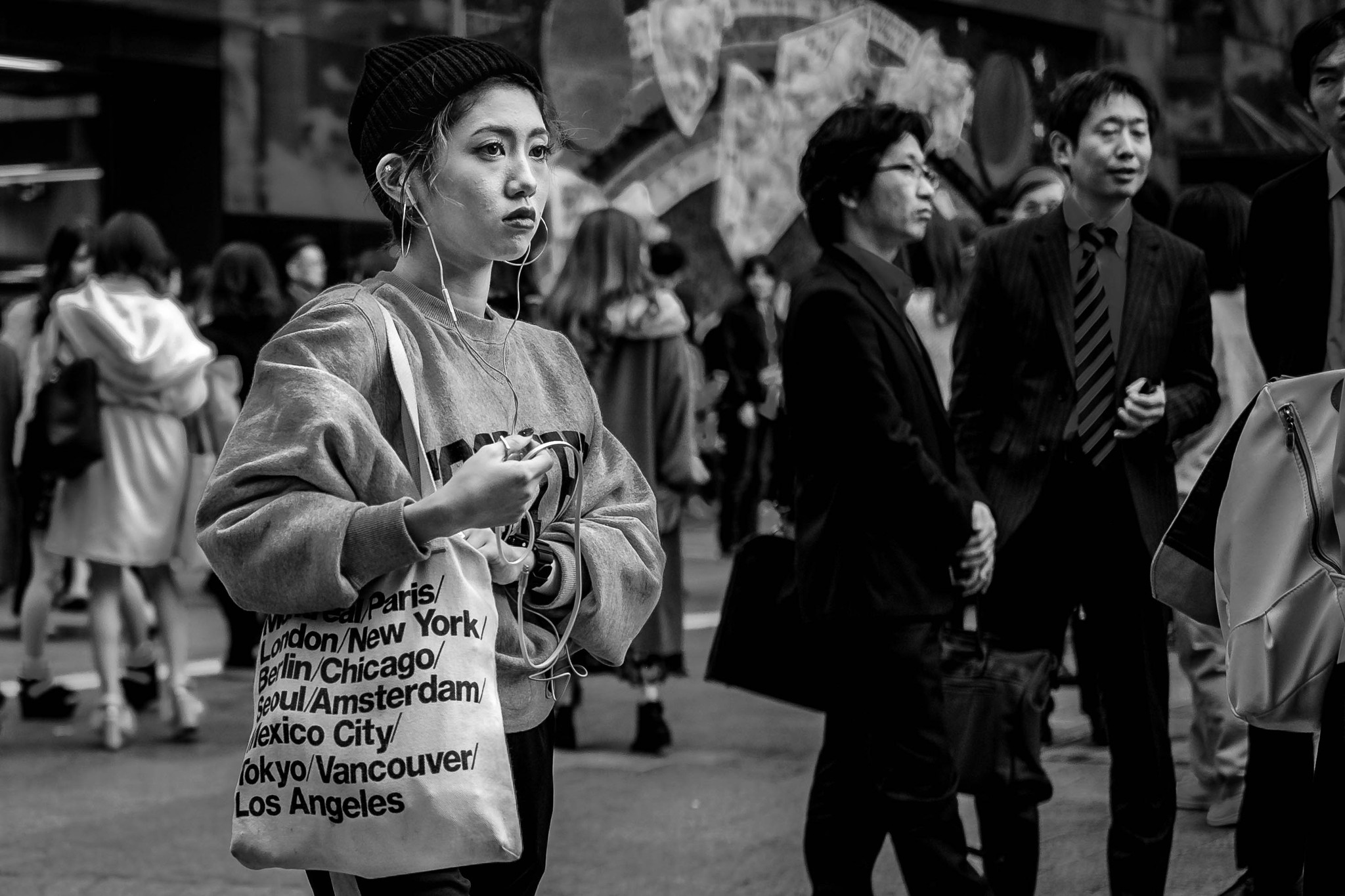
[228,296,523,880]
[943,633,1054,800]
[35,357,104,480]
[705,533,828,713]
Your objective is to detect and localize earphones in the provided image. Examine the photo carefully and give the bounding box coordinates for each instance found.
[399,174,416,205]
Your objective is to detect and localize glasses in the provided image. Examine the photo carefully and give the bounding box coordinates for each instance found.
[876,163,936,184]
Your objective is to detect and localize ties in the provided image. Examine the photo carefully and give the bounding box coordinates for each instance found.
[1073,229,1118,466]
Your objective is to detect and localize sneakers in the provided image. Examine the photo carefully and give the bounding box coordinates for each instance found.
[1176,772,1247,826]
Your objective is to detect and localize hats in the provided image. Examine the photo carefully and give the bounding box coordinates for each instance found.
[348,34,544,221]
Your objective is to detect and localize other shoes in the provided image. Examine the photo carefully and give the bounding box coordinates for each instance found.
[577,644,688,686]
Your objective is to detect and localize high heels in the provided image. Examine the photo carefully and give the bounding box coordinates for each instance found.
[621,706,668,758]
[553,701,579,749]
[120,660,162,712]
[166,681,207,733]
[16,676,78,720]
[91,695,135,747]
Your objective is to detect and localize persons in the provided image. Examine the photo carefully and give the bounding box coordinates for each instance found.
[946,68,1220,896]
[194,34,667,896]
[0,10,1345,896]
[777,102,998,896]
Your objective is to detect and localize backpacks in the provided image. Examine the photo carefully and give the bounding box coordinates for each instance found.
[1149,368,1345,738]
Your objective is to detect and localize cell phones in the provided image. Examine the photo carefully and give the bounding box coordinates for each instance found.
[1138,378,1161,395]
